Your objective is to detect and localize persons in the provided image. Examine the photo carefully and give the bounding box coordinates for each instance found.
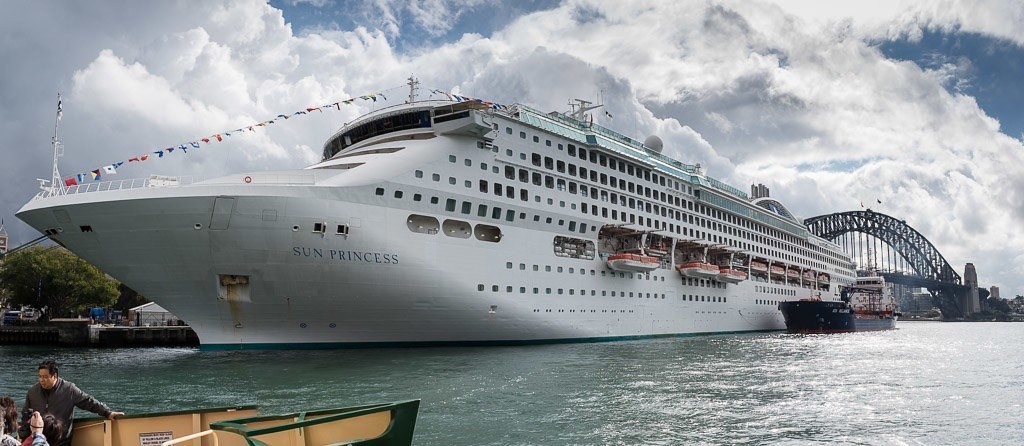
[21,360,124,446]
[0,397,50,446]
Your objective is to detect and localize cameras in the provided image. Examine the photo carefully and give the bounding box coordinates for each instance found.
[21,408,35,424]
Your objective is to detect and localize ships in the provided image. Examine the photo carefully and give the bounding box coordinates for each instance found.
[13,83,861,352]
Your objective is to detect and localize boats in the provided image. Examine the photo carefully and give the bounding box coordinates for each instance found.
[745,260,831,286]
[677,262,721,278]
[715,269,747,284]
[606,253,660,273]
[777,268,900,332]
